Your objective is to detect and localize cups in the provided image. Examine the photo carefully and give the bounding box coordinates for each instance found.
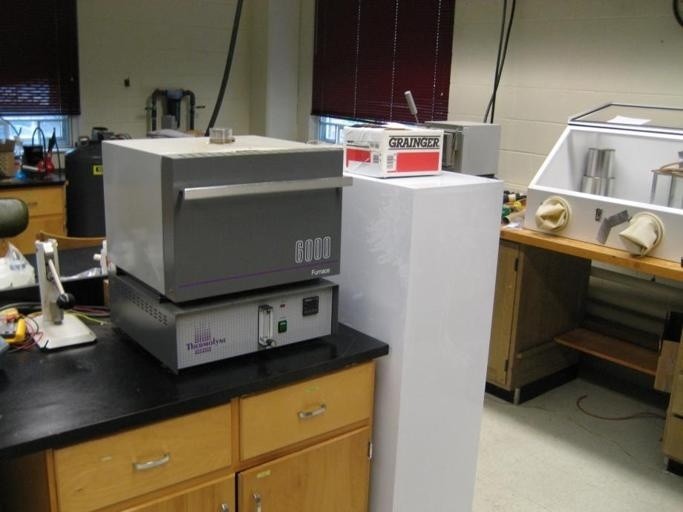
[208,127,233,144]
[582,145,617,199]
[22,145,43,178]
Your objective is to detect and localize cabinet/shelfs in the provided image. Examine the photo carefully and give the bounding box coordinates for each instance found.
[0,170,70,258]
[0,308,390,511]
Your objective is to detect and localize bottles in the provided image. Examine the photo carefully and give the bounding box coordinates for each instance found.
[502,194,527,219]
[13,138,27,182]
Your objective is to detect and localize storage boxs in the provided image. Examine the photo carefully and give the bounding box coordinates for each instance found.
[341,123,444,178]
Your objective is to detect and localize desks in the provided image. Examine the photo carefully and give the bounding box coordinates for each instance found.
[482,226,682,478]
